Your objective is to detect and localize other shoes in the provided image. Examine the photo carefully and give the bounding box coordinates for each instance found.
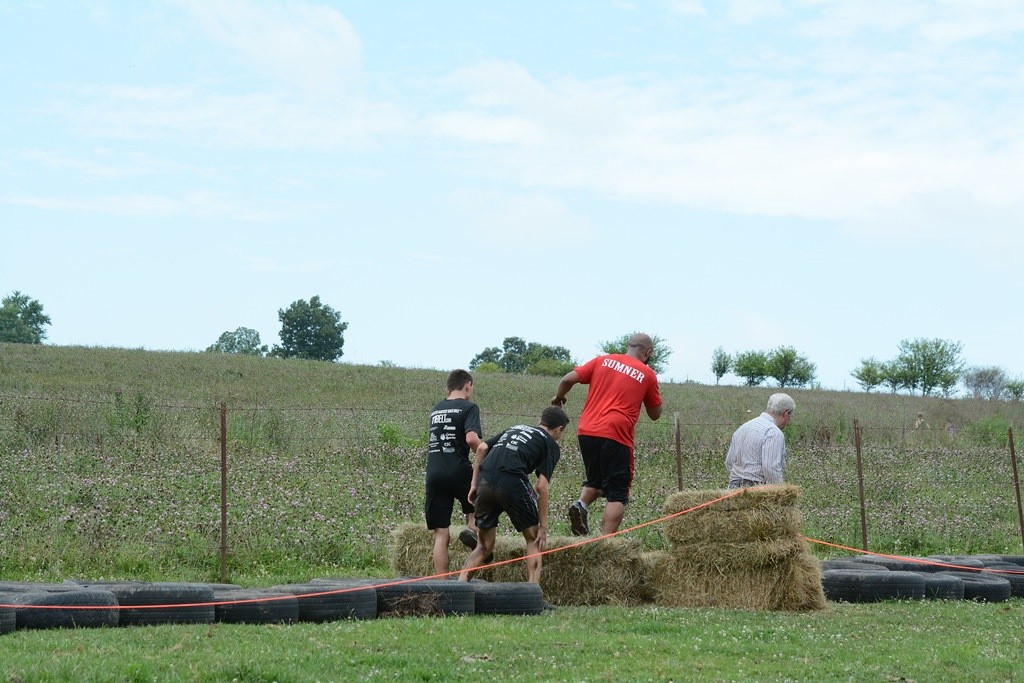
[458,528,493,563]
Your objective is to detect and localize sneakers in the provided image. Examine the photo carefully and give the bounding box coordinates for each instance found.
[568,501,589,536]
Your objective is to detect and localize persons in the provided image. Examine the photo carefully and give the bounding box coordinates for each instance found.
[456,406,569,610]
[425,370,494,580]
[943,417,958,447]
[915,412,931,429]
[551,333,663,539]
[725,393,796,490]
